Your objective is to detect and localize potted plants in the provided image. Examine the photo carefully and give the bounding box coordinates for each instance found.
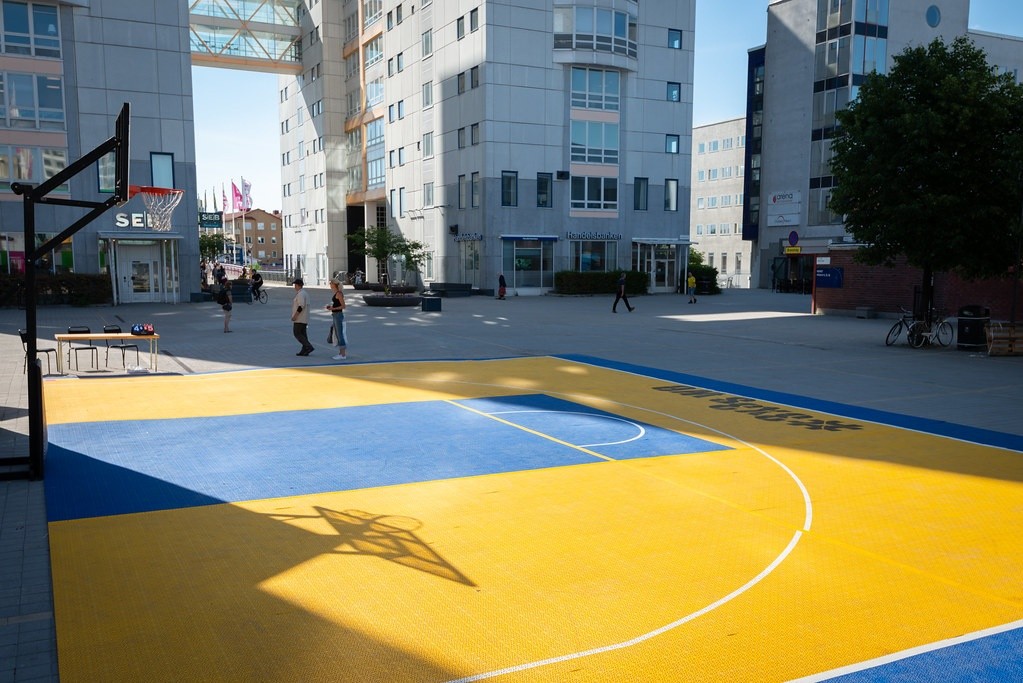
[498,274,507,300]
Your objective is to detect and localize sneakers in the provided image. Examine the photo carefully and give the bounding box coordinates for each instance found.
[332,354,346,360]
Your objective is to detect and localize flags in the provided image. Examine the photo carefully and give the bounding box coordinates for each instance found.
[205,179,253,212]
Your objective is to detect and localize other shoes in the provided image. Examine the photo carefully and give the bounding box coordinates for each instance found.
[688,300,693,303]
[302,345,315,355]
[693,299,697,304]
[612,310,618,313]
[296,350,309,356]
[224,330,232,333]
[629,307,635,312]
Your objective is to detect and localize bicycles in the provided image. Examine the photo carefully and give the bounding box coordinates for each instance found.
[244,283,268,305]
[885,303,953,348]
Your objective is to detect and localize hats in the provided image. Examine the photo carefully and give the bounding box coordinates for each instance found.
[294,279,303,285]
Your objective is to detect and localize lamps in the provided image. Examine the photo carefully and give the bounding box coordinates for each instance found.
[454,233,482,243]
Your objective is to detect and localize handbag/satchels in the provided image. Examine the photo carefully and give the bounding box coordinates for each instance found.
[327,322,348,346]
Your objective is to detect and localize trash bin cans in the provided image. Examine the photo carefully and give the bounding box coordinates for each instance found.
[956,305,991,352]
[381,274,387,284]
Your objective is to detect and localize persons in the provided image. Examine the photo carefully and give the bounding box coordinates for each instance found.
[200,262,225,284]
[220,278,232,333]
[292,279,314,356]
[326,278,348,359]
[688,273,695,304]
[612,273,635,313]
[496,272,506,300]
[242,266,263,300]
[355,268,363,283]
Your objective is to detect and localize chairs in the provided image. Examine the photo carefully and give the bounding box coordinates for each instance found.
[68,325,98,372]
[104,325,139,369]
[18,329,59,376]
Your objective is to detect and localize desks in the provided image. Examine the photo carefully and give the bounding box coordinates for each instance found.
[55,332,160,374]
